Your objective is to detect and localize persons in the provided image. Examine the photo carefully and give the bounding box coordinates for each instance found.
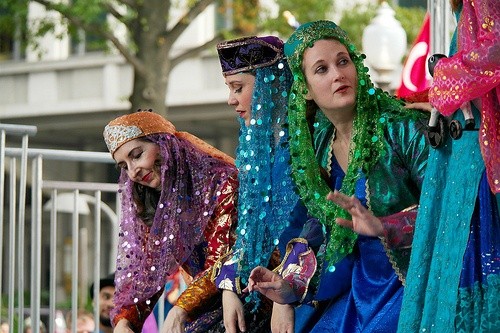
[185,36,326,333]
[397,0,500,333]
[0,278,113,333]
[240,19,431,333]
[102,108,239,333]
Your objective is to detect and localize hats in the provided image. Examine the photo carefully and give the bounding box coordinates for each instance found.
[216,35,284,76]
[284,20,354,72]
[102,110,176,158]
[89,273,115,299]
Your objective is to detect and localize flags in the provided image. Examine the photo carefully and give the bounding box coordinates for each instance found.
[393,15,430,103]
